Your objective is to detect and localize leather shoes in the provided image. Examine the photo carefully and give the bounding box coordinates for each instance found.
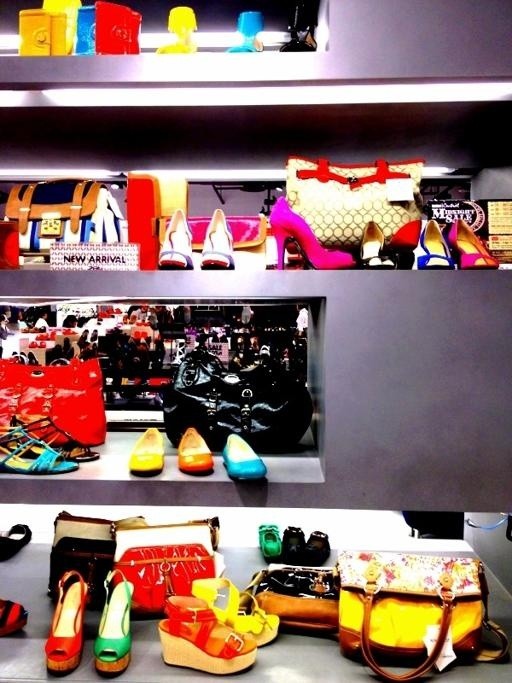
[200,209,235,269]
[129,427,165,474]
[0,524,32,560]
[221,433,267,480]
[158,210,193,269]
[258,525,330,565]
[177,426,215,472]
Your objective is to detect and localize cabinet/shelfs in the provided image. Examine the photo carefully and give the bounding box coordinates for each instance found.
[0,0,512,680]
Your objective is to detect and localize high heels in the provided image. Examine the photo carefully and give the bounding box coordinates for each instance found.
[158,578,280,675]
[270,196,357,269]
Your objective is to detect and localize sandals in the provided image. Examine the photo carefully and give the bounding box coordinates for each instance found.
[45,569,89,672]
[0,598,28,635]
[226,11,265,51]
[94,568,134,674]
[280,7,317,51]
[1,415,100,475]
[417,219,454,270]
[360,220,400,269]
[448,218,499,268]
[156,7,198,54]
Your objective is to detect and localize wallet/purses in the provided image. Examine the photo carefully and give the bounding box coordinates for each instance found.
[17,2,142,56]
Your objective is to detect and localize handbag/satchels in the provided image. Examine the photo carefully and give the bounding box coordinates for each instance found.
[155,214,269,270]
[0,356,107,446]
[165,350,314,453]
[239,562,339,633]
[49,510,227,616]
[336,549,509,682]
[5,178,123,255]
[285,154,425,248]
